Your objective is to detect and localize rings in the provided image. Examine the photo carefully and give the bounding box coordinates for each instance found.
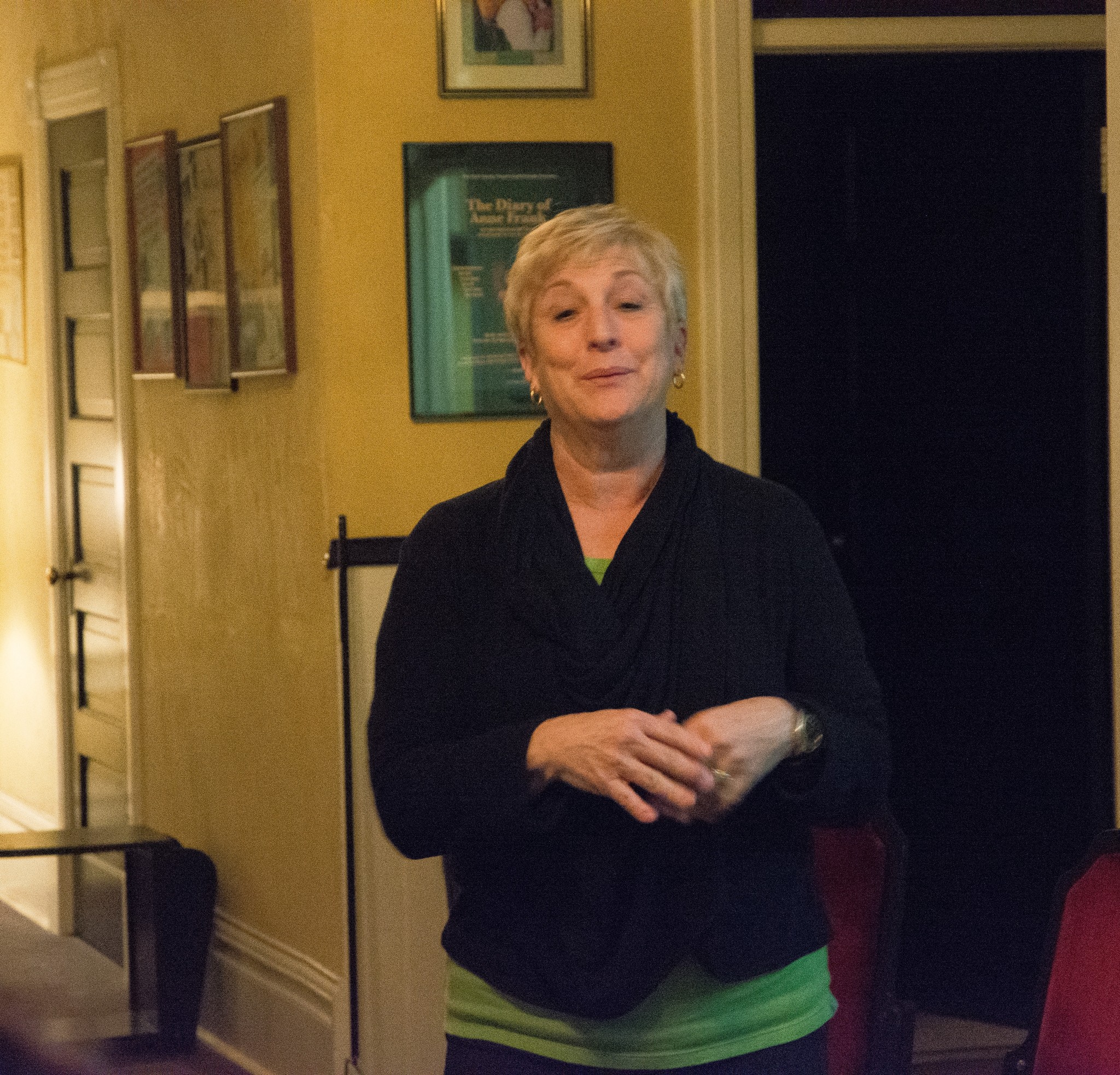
[710,768,731,780]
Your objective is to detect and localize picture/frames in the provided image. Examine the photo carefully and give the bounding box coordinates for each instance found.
[170,133,240,395]
[121,128,184,381]
[217,96,297,380]
[433,0,596,99]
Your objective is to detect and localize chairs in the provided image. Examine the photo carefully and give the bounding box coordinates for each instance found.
[1003,823,1120,1075]
[806,808,917,1074]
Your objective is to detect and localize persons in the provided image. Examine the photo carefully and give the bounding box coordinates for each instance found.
[370,204,889,1075]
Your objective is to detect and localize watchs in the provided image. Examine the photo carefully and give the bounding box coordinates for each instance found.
[783,698,823,754]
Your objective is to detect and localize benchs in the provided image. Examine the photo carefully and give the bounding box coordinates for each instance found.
[1,823,217,1058]
